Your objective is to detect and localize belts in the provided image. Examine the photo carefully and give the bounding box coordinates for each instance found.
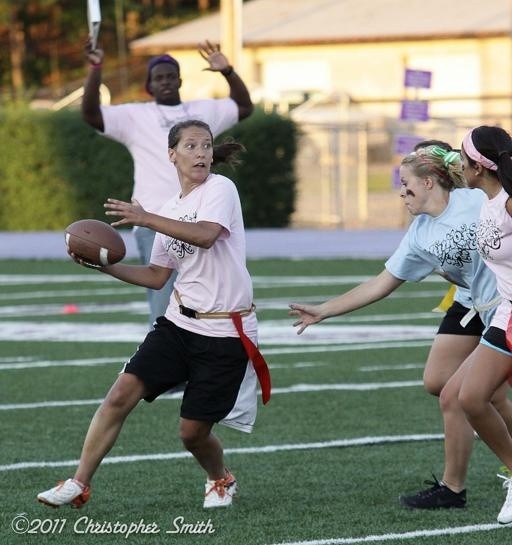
[170,286,256,325]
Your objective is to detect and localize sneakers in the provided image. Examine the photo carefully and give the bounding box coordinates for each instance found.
[35,478,91,510]
[397,476,468,510]
[494,470,511,525]
[202,470,238,511]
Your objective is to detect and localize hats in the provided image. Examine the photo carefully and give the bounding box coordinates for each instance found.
[145,54,180,91]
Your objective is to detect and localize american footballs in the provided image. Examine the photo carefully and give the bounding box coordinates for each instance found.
[64,218,126,266]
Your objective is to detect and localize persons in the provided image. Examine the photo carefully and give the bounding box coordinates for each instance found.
[82,34,252,328]
[37,120,257,509]
